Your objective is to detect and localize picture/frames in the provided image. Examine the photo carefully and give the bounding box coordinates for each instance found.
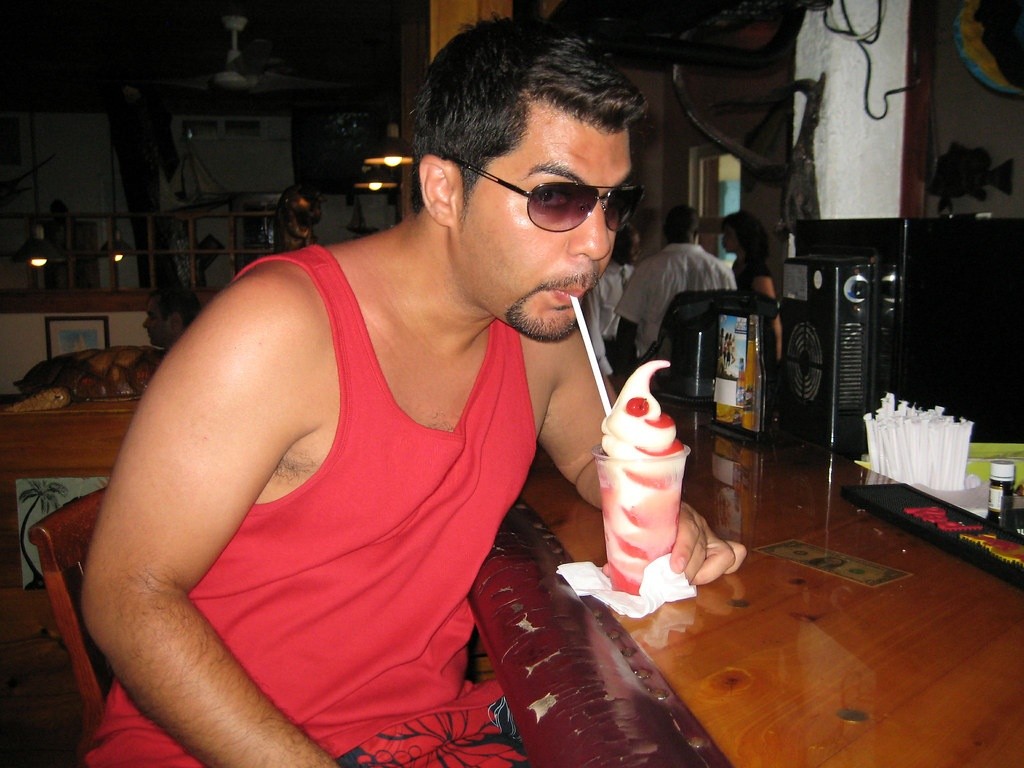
[44,315,109,360]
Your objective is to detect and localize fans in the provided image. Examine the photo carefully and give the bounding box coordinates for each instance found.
[143,16,346,92]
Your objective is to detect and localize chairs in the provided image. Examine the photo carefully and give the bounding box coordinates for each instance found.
[30,483,115,734]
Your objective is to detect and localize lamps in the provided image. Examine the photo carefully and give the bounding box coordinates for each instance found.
[10,82,71,268]
[362,91,416,168]
[99,123,133,261]
[352,113,398,192]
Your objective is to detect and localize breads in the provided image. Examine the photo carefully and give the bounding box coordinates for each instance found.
[17,344,158,401]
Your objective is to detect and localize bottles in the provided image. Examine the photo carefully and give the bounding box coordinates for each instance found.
[989,461,1016,521]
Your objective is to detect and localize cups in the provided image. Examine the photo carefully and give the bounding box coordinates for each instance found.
[593,442,690,595]
[1003,496,1024,537]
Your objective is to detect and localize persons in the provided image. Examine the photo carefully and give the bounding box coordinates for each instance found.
[77,15,746,768]
[583,204,783,431]
[142,286,200,350]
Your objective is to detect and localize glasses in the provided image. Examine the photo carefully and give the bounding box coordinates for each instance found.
[443,154,644,233]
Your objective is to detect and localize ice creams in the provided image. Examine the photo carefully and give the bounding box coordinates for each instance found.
[599,359,689,596]
